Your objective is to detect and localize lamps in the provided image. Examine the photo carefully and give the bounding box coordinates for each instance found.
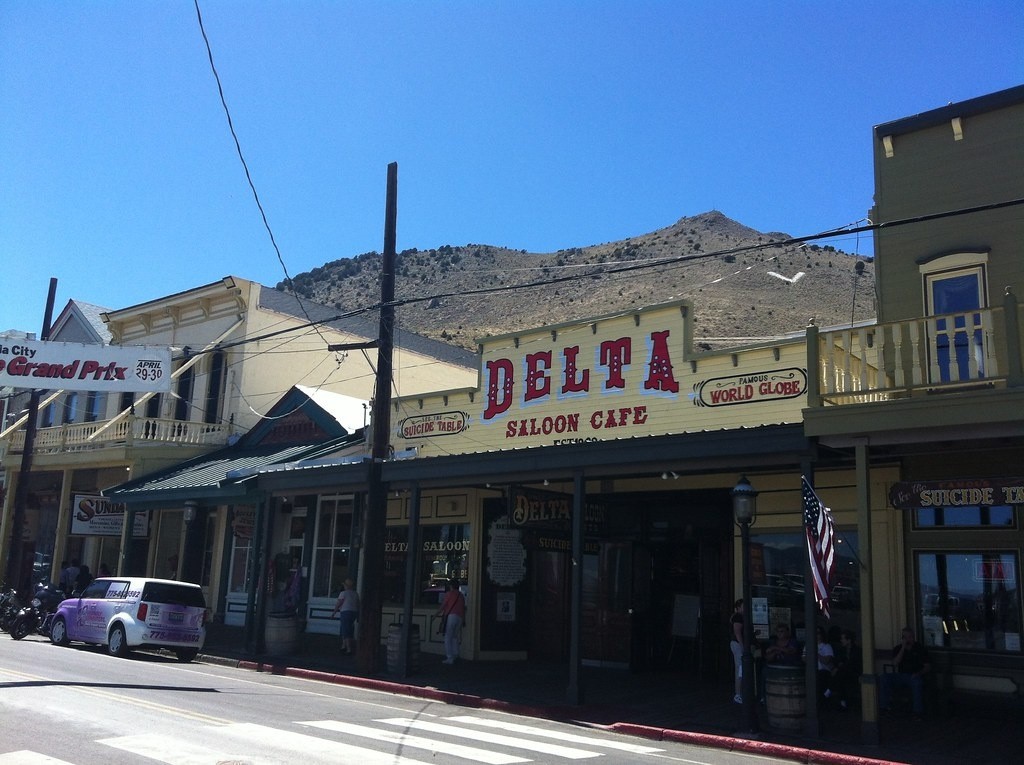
[184,499,199,525]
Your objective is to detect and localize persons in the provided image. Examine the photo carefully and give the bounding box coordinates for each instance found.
[330,578,360,656]
[431,577,465,666]
[727,596,932,721]
[60,558,113,598]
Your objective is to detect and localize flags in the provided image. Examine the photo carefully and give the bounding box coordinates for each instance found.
[800,474,839,616]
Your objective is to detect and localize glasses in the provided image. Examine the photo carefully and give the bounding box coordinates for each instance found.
[776,630,786,632]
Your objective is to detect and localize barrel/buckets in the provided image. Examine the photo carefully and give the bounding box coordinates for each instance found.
[764,664,807,729]
[263,612,299,659]
[386,623,421,675]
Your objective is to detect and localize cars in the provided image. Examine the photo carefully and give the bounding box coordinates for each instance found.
[49,577,207,663]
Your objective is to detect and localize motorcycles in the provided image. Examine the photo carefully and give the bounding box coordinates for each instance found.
[10,551,68,640]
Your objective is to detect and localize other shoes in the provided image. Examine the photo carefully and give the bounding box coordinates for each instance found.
[879,709,888,717]
[338,645,352,653]
[734,694,744,703]
[912,715,923,721]
[442,660,454,664]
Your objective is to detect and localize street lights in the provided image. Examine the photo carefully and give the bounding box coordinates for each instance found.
[183,500,198,583]
[730,474,759,735]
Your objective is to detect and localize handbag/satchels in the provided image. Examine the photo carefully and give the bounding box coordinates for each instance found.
[437,615,447,636]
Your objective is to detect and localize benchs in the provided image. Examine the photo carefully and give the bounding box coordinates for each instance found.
[889,671,1018,718]
